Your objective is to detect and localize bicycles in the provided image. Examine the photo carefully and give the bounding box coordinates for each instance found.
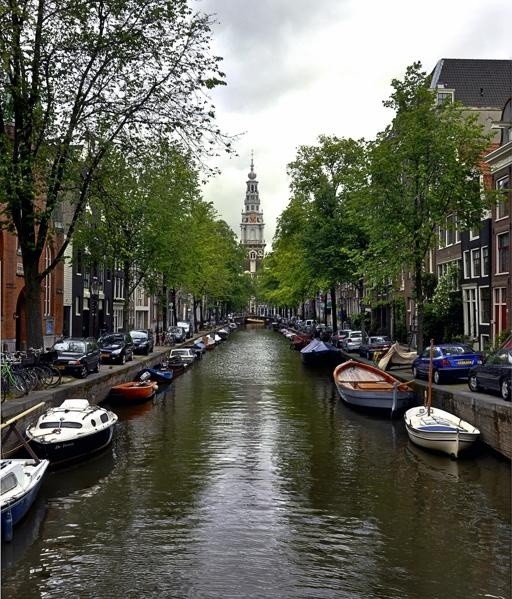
[1,347,62,404]
[157,331,176,348]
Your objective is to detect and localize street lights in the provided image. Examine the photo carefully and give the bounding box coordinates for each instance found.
[339,296,347,330]
[76,270,111,374]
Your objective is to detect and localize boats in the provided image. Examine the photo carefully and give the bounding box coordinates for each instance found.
[111,380,159,402]
[301,338,340,364]
[169,348,198,367]
[404,338,480,457]
[333,358,413,417]
[277,326,311,351]
[404,440,482,483]
[1,455,50,542]
[24,397,118,468]
[134,363,174,384]
[47,451,118,512]
[2,507,50,574]
[156,355,193,376]
[185,321,241,359]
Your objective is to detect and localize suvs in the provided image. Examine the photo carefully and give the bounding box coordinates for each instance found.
[177,320,193,338]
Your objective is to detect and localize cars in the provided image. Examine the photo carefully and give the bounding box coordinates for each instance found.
[98,333,135,365]
[46,336,102,379]
[128,329,154,355]
[165,326,185,342]
[468,349,512,400]
[411,343,483,384]
[276,319,372,354]
[359,336,394,360]
